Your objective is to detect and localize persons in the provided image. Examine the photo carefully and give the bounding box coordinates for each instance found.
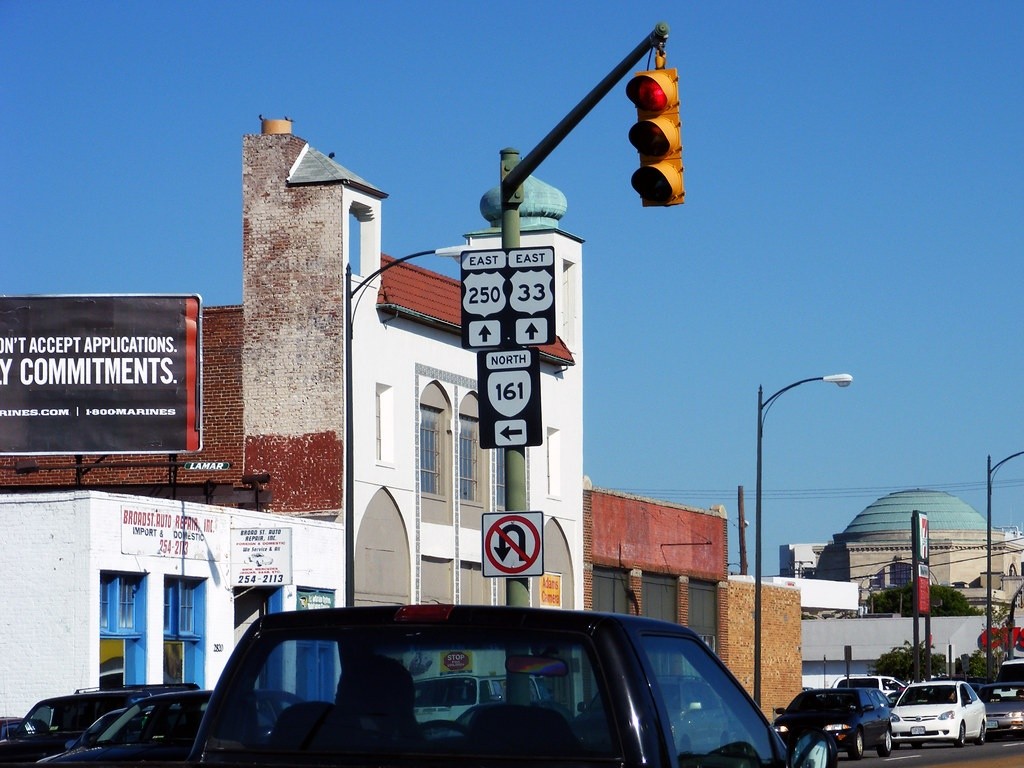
[948,688,957,702]
[1016,690,1024,698]
[337,657,415,740]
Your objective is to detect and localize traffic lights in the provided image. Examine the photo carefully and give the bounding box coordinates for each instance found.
[626,67,686,207]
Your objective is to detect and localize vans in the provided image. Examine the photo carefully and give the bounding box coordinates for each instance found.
[990,657,1024,701]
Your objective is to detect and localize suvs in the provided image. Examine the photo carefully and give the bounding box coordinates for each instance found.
[178,603,838,768]
[39,688,308,763]
[0,682,202,767]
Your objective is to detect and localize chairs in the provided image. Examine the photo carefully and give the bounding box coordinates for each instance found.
[467,703,592,759]
[986,694,1003,702]
[333,654,430,755]
[265,700,366,755]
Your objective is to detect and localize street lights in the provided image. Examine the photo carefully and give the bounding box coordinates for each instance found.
[345,245,493,608]
[753,373,853,709]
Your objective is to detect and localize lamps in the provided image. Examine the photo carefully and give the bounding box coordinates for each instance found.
[242,474,270,512]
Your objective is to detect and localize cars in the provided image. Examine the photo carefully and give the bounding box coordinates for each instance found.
[35,704,208,764]
[772,687,892,762]
[0,716,53,742]
[974,681,1024,743]
[890,680,988,750]
[816,675,990,707]
